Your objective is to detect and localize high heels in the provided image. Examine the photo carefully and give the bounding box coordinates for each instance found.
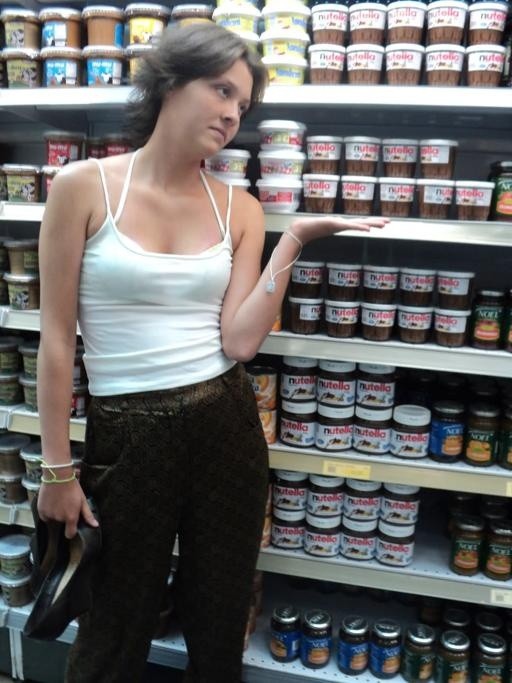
[21,490,102,643]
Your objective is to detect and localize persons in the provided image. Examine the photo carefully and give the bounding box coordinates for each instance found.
[35,18,394,682]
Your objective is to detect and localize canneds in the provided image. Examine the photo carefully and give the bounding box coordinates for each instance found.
[242,570,512,683]
[244,355,511,473]
[489,161,512,221]
[270,258,512,353]
[262,471,512,582]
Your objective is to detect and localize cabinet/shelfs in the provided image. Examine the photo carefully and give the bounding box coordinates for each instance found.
[0,0,510,683]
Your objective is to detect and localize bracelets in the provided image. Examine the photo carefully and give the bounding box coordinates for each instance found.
[264,229,303,294]
[39,458,77,484]
